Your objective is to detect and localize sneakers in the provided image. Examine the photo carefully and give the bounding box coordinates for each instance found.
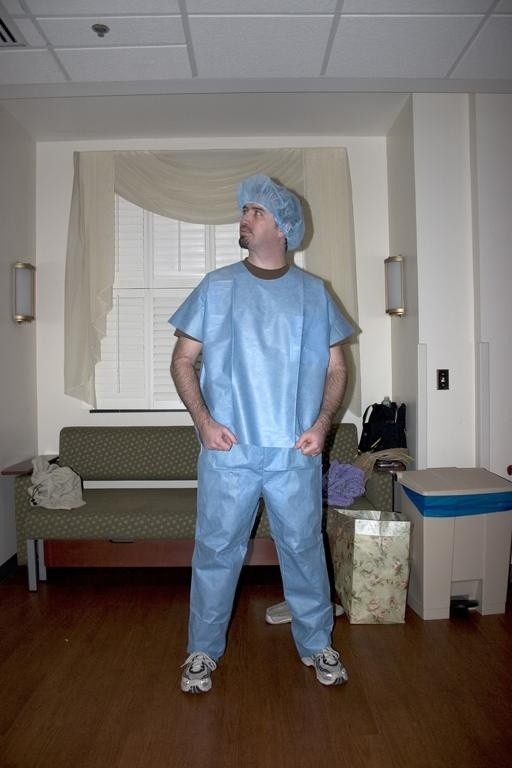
[265,600,291,624]
[301,645,348,686]
[182,651,217,692]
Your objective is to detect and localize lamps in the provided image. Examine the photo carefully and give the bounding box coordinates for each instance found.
[385,255,406,318]
[12,261,35,325]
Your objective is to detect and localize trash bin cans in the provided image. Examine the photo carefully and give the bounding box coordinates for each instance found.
[394,465,512,621]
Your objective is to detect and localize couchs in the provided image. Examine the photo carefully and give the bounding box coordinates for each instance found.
[0,423,406,592]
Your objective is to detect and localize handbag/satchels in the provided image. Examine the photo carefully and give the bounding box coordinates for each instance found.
[359,401,408,453]
[27,458,87,510]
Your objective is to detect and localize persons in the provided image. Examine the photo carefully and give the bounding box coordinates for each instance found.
[164,172,364,697]
